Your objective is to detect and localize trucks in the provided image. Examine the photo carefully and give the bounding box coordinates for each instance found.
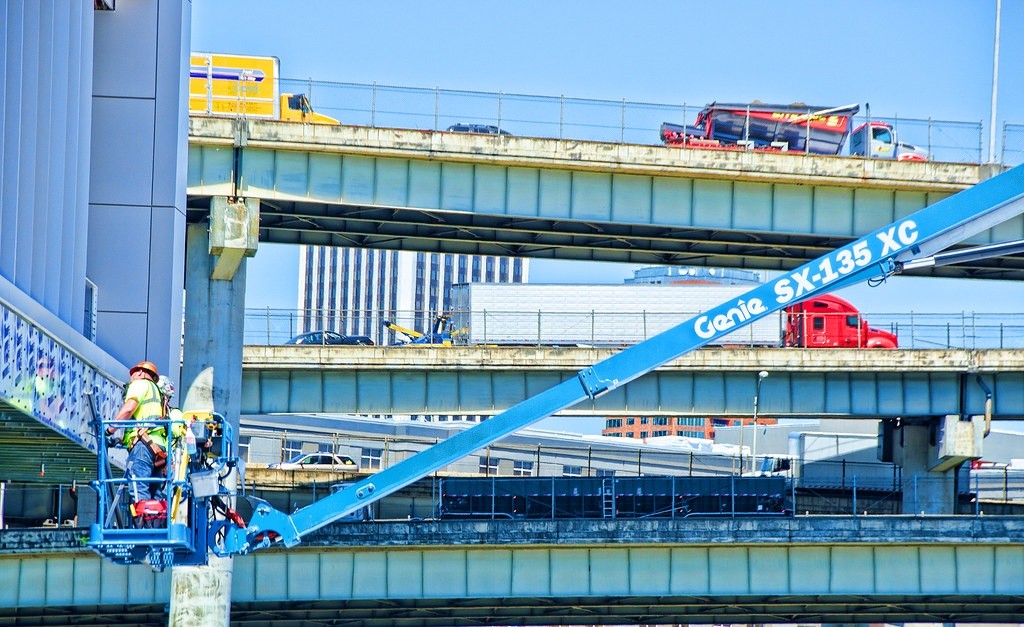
[185,50,339,132]
[439,274,902,354]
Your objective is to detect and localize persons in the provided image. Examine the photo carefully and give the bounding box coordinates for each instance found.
[104,361,186,528]
[10,356,69,431]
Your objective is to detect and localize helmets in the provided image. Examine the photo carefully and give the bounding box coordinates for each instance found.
[129,362,159,383]
[156,375,175,398]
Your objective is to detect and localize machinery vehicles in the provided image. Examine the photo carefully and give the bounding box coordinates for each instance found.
[661,96,937,165]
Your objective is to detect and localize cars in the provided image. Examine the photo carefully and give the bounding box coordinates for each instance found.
[271,452,359,471]
[443,120,512,141]
